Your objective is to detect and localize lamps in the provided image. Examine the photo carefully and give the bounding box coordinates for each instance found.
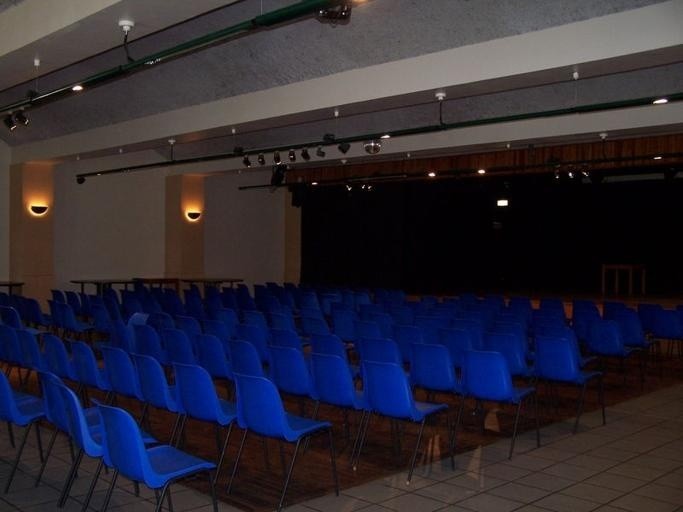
[316,2,351,23]
[241,145,325,167]
[553,164,590,179]
[343,179,373,192]
[30,205,48,214]
[3,110,27,130]
[187,213,199,219]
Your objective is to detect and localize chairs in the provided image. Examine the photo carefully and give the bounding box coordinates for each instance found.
[0,282,681,510]
